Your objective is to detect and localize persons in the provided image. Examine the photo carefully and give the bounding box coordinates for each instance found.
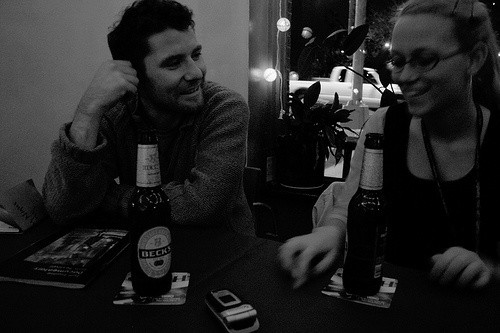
[275,0,500,295]
[43,1,257,235]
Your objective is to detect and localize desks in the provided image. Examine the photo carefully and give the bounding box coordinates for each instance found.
[0,217,500,332]
[254,191,323,242]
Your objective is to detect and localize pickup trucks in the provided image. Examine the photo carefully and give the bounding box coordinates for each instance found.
[289,66,407,109]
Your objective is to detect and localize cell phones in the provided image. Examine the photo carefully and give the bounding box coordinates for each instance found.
[205,290,259,333]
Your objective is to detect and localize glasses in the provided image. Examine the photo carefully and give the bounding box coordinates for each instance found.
[385,46,467,73]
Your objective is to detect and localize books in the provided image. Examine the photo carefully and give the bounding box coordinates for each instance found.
[0,225,132,288]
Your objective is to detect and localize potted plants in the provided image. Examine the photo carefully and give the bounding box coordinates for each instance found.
[263,80,361,199]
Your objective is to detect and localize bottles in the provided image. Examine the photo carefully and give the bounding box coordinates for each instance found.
[126,131,173,298]
[343,132,388,297]
